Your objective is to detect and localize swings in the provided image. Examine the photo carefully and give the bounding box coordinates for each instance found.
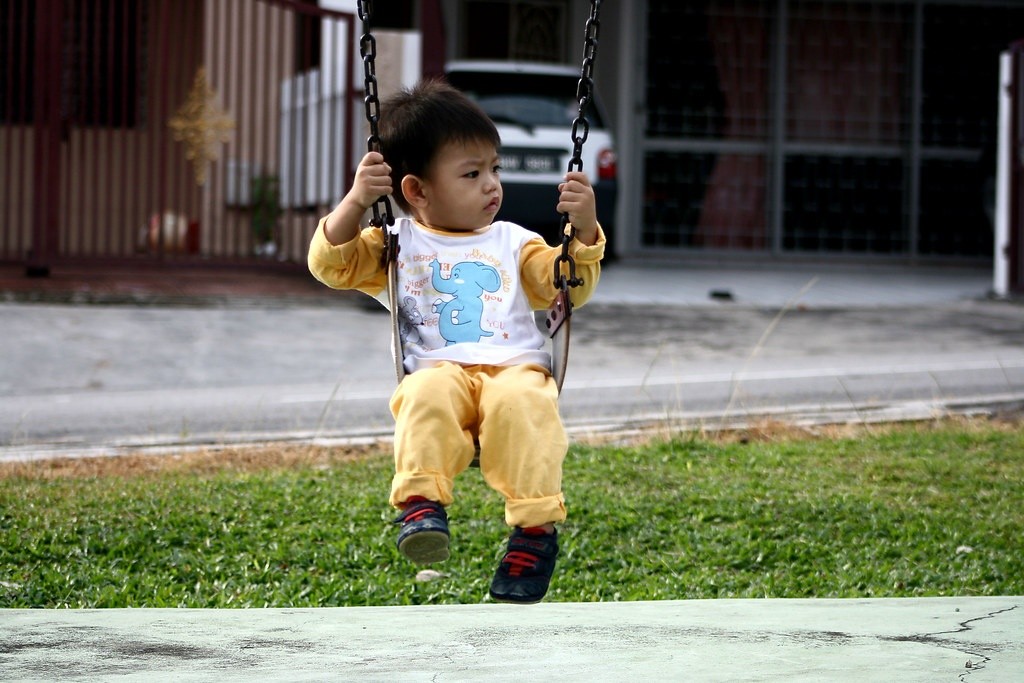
[356,0,601,469]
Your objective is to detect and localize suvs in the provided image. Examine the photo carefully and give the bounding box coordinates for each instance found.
[439,58,618,268]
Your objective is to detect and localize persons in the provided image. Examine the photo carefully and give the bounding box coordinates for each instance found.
[306,80,607,603]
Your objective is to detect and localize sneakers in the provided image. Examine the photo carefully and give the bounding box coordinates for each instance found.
[393,499,451,564]
[489,525,559,605]
